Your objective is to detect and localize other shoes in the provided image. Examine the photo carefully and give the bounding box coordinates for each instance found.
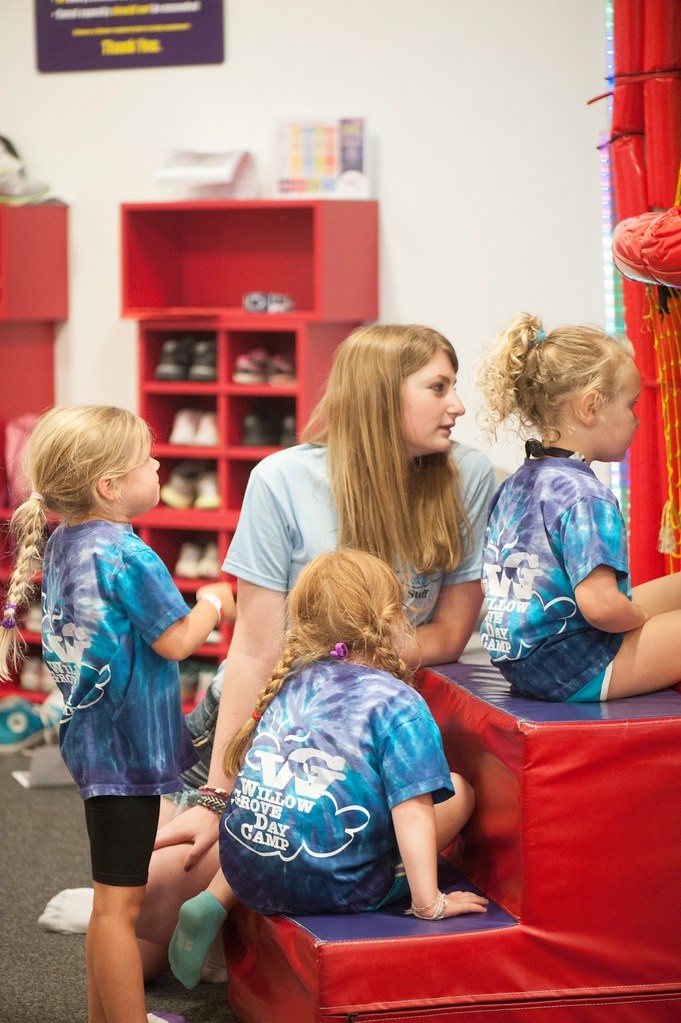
[167,410,219,447]
[25,607,47,631]
[238,410,294,446]
[175,542,220,580]
[233,346,295,385]
[159,462,222,507]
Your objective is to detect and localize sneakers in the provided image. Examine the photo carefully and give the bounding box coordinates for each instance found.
[22,654,66,695]
[178,662,217,693]
[152,336,218,383]
[0,699,63,754]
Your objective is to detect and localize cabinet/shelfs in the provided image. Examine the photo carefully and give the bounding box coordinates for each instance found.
[116,194,381,665]
[0,505,65,702]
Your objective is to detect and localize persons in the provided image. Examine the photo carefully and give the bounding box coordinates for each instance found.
[0,405,238,1023]
[478,313,681,702]
[219,546,491,922]
[134,324,493,983]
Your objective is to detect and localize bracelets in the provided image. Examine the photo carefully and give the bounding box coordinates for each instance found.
[404,888,445,921]
[198,589,225,624]
[184,784,230,814]
